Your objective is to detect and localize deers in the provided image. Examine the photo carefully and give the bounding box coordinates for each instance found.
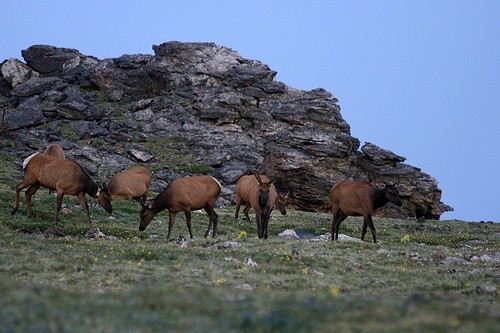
[43,143,66,196]
[139,176,222,239]
[330,179,402,244]
[249,169,278,239]
[11,152,113,226]
[235,175,290,223]
[108,166,152,203]
